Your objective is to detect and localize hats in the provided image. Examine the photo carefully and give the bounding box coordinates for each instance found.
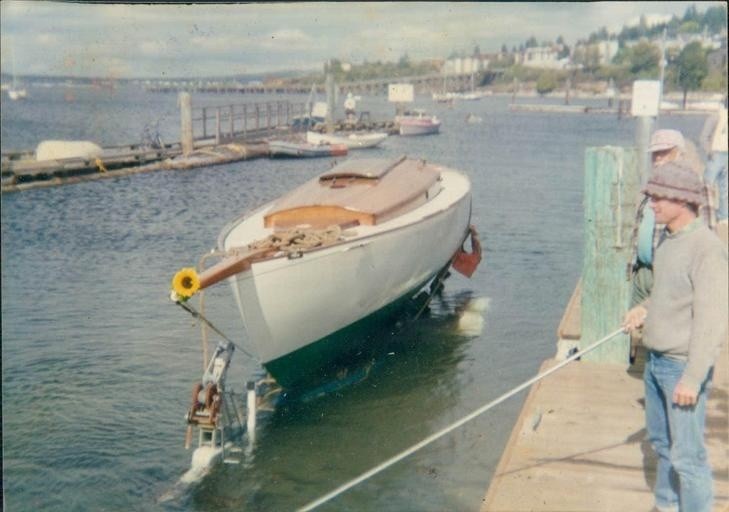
[639,158,705,206]
[646,128,686,153]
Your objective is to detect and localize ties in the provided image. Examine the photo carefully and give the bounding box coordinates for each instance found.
[631,195,649,266]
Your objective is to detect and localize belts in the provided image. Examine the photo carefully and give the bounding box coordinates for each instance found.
[635,256,654,272]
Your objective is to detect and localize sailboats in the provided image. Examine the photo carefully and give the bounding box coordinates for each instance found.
[2,29,26,100]
[433,54,492,103]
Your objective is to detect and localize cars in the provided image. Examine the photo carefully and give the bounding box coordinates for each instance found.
[293,102,328,128]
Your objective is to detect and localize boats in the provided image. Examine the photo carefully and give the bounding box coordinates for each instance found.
[267,138,350,154]
[398,114,441,137]
[169,154,475,401]
[395,104,427,122]
[306,130,388,150]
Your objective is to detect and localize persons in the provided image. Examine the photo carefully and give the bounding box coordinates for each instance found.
[622,160,729,512]
[626,130,687,369]
[700,95,728,223]
